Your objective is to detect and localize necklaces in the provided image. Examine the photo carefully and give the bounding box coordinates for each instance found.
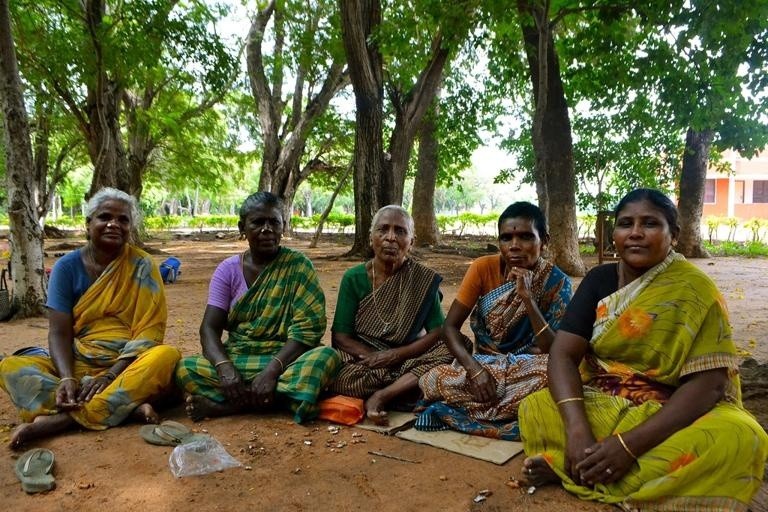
[370,261,403,331]
[86,243,103,271]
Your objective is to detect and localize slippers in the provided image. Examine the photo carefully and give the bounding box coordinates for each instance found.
[141,420,213,452]
[17,448,56,492]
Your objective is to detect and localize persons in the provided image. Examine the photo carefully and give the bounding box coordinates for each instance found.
[411,201,573,442]
[175,193,340,422]
[0,187,182,450]
[330,205,471,426]
[519,187,768,510]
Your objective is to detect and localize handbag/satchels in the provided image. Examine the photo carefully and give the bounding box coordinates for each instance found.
[0,271,10,321]
[318,394,366,426]
[160,256,181,283]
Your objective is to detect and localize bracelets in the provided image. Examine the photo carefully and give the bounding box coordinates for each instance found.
[616,431,640,459]
[531,324,550,339]
[273,354,286,370]
[468,367,486,381]
[59,377,80,382]
[553,397,588,404]
[214,359,233,367]
[100,371,119,380]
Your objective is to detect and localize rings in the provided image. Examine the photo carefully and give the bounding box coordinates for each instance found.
[606,469,612,475]
[514,266,517,270]
